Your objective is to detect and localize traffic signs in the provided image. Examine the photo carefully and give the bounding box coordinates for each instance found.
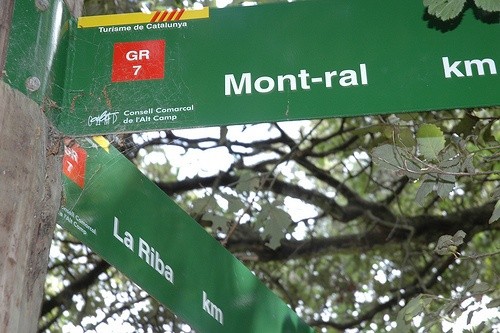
[51,122,319,332]
[57,0,500,139]
